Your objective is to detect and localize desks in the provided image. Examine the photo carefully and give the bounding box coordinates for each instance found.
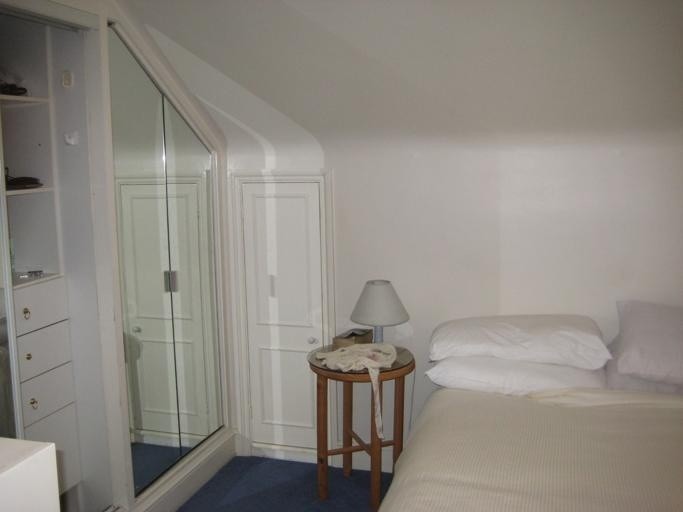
[306,344,415,511]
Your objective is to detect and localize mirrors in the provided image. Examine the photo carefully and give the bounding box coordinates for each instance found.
[108,24,224,498]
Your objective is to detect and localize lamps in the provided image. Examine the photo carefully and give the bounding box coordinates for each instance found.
[349,279,410,344]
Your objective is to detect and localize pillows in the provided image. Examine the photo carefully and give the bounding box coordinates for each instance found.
[424,300,683,395]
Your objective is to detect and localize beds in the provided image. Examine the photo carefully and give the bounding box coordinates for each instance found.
[378,387,682,510]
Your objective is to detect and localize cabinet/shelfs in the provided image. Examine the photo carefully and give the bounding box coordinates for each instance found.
[0,0,106,512]
